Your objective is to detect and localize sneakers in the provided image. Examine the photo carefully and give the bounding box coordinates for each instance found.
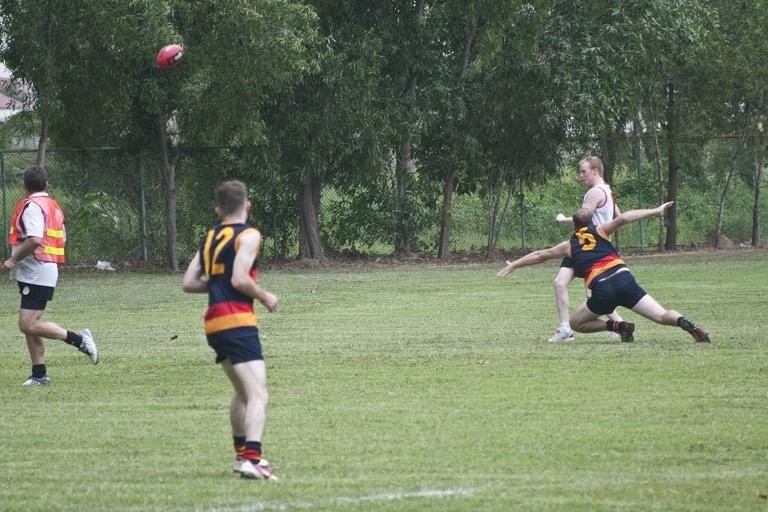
[77,329,98,365]
[233,458,277,480]
[691,326,708,342]
[22,376,50,385]
[548,328,575,342]
[609,322,634,342]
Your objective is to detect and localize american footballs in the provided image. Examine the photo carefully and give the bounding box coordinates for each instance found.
[157,44,184,66]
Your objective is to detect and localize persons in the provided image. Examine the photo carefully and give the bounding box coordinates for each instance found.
[495,201,713,344]
[182,180,284,481]
[549,155,626,342]
[1,166,98,386]
